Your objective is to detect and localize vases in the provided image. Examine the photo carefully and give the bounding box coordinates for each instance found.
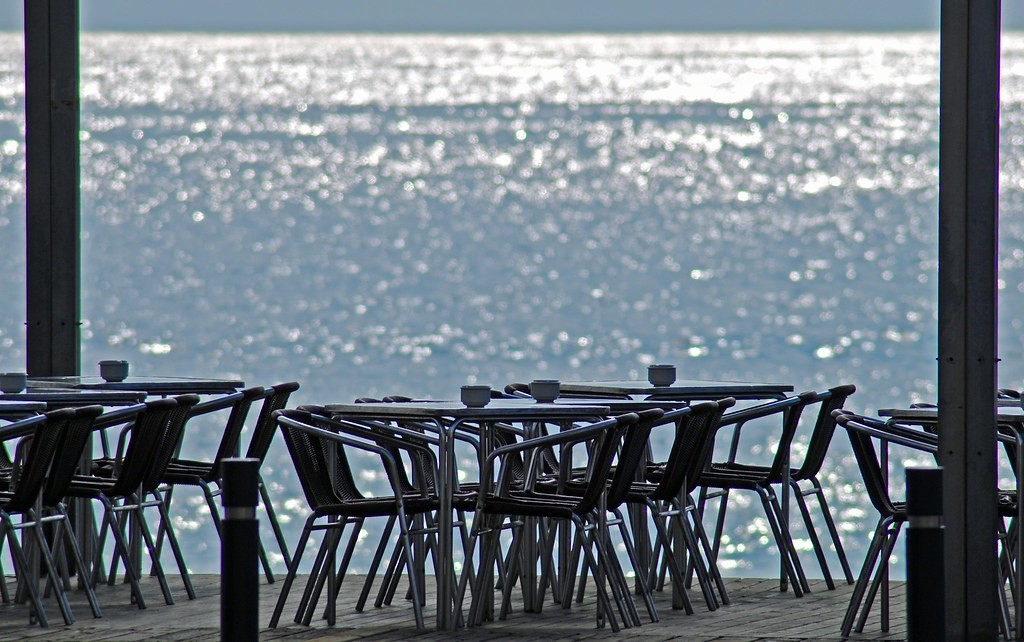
[0,373,27,393]
[99,360,129,381]
[647,364,676,387]
[459,386,491,408]
[528,380,559,402]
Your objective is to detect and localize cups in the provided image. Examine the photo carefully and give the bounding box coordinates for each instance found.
[460,385,492,408]
[647,364,677,387]
[529,380,560,402]
[97,360,129,382]
[0,373,29,393]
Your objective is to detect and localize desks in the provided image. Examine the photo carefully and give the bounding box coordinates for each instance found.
[558,383,795,597]
[25,373,247,596]
[889,406,1024,642]
[325,400,609,628]
[425,396,687,608]
[0,386,148,611]
[0,400,48,625]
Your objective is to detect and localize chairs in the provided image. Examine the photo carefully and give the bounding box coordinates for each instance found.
[0,382,1024,642]
